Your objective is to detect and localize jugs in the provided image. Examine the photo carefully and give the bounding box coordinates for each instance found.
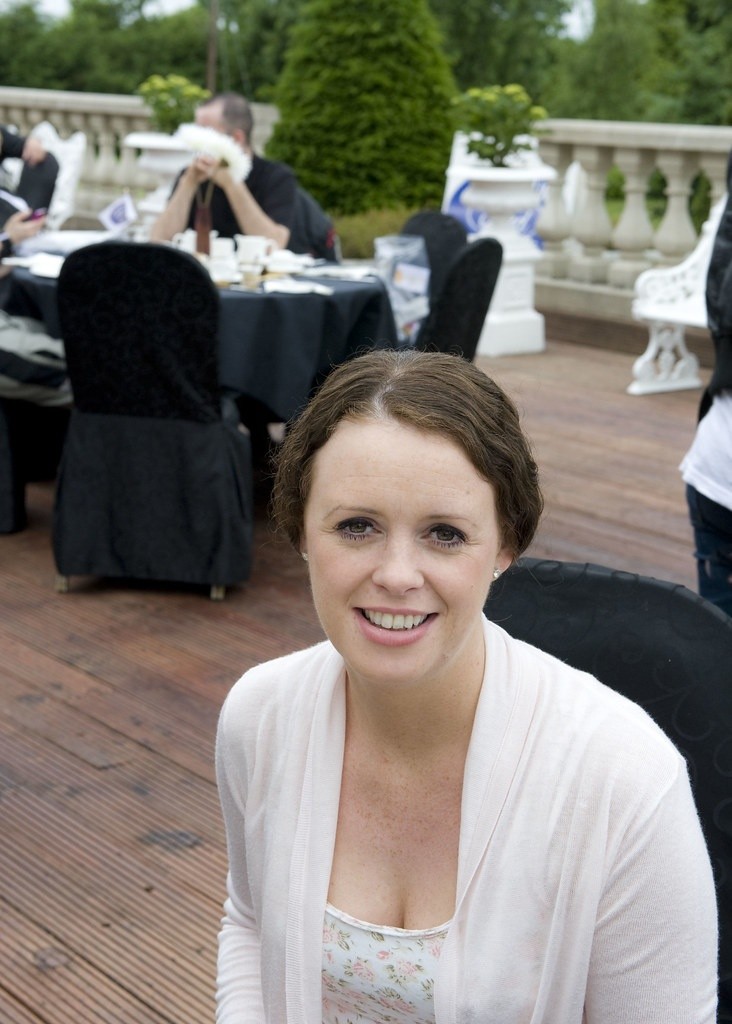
[234,233,277,266]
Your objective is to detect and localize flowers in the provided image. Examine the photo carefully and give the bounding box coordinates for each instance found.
[179,119,252,255]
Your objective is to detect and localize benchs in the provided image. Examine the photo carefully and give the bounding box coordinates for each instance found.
[628,192,726,397]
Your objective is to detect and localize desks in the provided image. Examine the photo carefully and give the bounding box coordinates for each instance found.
[14,232,400,429]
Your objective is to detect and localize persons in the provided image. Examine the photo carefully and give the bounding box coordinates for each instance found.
[214,350,717,1024]
[679,148,732,614]
[146,92,310,259]
[0,113,48,279]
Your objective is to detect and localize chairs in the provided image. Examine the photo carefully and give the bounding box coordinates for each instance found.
[395,209,509,360]
[494,559,732,1024]
[0,404,65,535]
[51,242,247,604]
[46,132,88,229]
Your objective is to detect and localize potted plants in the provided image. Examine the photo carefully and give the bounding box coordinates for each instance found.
[439,80,560,356]
[119,72,213,220]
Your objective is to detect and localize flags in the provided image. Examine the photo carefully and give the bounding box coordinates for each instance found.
[97,194,138,230]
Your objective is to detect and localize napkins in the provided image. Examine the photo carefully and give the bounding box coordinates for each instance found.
[263,279,333,298]
[305,265,376,279]
[3,253,65,276]
